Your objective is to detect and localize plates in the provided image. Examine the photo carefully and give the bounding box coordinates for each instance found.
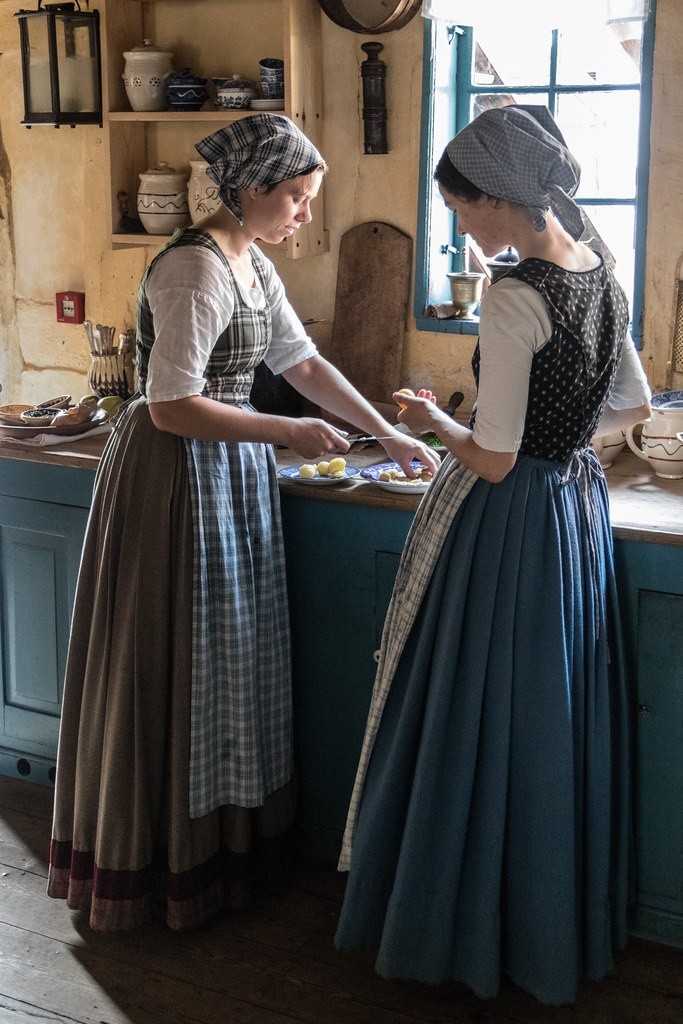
[360,462,438,495]
[277,465,360,486]
[651,390,683,411]
[250,99,285,111]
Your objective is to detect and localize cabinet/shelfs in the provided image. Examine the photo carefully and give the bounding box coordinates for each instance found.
[1,458,682,920]
[98,0,292,239]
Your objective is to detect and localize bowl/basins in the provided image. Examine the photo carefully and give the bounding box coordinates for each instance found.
[216,74,257,108]
[259,58,284,99]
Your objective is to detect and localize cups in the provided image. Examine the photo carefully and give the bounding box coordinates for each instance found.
[446,272,487,319]
[626,411,683,479]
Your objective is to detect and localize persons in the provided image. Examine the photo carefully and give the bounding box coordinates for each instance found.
[45,112,440,937]
[332,105,651,1008]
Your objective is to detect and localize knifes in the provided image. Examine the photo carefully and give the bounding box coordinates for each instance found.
[344,436,396,441]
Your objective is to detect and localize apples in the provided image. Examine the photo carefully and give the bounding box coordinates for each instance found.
[80,394,124,419]
